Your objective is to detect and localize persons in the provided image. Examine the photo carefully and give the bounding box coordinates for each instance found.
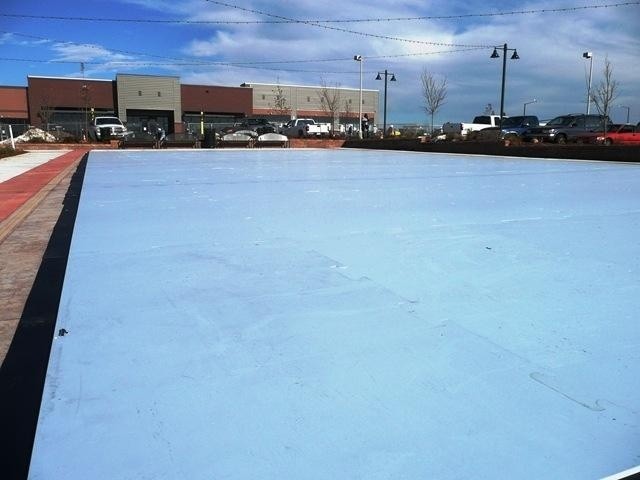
[154,127,258,149]
[362,113,370,138]
[372,124,378,134]
[348,125,352,139]
[387,124,394,136]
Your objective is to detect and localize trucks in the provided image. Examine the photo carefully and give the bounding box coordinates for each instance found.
[479,114,550,143]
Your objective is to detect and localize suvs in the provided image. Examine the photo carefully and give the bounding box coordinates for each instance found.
[88,115,128,143]
[526,111,614,144]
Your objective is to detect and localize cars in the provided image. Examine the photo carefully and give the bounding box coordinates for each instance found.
[575,121,640,148]
[386,125,446,144]
[219,117,274,135]
[0,123,29,141]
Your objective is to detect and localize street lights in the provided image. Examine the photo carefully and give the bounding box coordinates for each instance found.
[353,53,364,139]
[488,42,521,133]
[582,51,594,115]
[619,104,631,125]
[523,98,538,116]
[374,68,398,136]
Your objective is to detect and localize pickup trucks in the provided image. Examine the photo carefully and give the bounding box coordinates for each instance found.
[438,114,510,141]
[280,118,334,140]
